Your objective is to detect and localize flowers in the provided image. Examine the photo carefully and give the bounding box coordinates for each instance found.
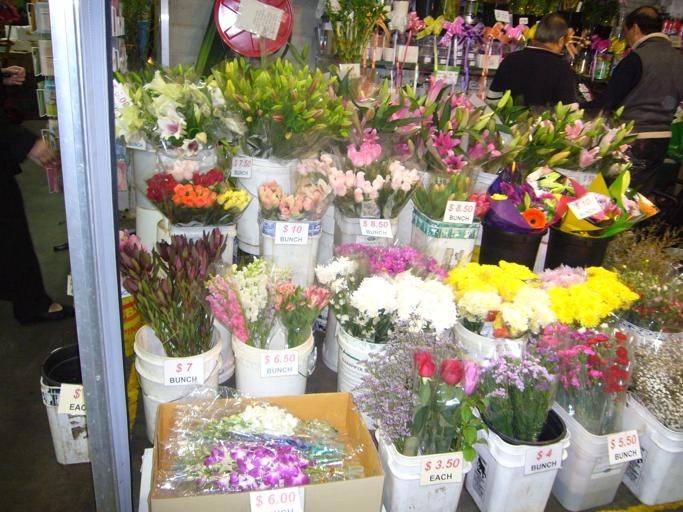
[315,154,422,220]
[470,353,559,442]
[141,169,254,227]
[319,0,389,65]
[338,268,457,336]
[314,244,449,298]
[518,101,640,173]
[423,80,516,171]
[213,56,352,159]
[603,221,683,332]
[541,266,640,328]
[486,160,587,231]
[113,56,234,156]
[410,172,489,220]
[155,403,365,498]
[259,163,335,223]
[116,229,229,358]
[537,323,630,435]
[336,78,437,165]
[444,254,556,339]
[353,314,489,464]
[634,337,682,433]
[203,257,331,350]
[552,168,662,236]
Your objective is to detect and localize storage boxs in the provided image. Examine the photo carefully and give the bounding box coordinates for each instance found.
[147,393,385,512]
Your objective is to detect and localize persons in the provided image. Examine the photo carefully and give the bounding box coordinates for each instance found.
[579,5,683,228]
[486,14,575,116]
[1,60,73,324]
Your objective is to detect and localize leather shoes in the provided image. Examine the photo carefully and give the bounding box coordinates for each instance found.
[17,303,73,326]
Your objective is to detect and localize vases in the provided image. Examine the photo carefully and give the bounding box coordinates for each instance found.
[480,407,567,445]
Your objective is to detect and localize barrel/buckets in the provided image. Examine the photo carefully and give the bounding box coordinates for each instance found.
[456,321,526,361]
[133,317,223,445]
[321,310,340,374]
[619,392,683,506]
[465,406,571,512]
[233,150,299,257]
[336,324,388,434]
[483,227,542,268]
[547,225,613,275]
[39,344,90,465]
[257,212,322,290]
[373,426,467,512]
[552,400,645,512]
[122,295,141,358]
[231,328,314,396]
[617,317,682,342]
[410,207,482,269]
[132,144,197,209]
[156,220,238,273]
[332,207,399,248]
